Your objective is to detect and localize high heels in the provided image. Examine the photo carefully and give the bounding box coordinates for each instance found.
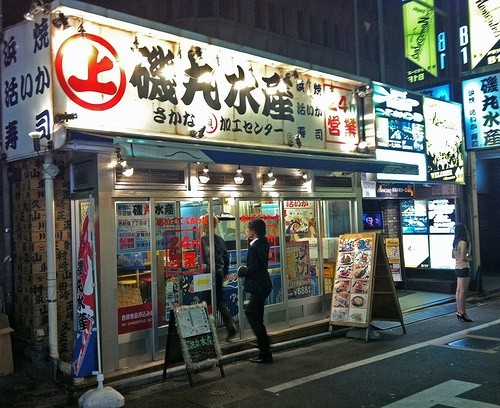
[456,312,473,322]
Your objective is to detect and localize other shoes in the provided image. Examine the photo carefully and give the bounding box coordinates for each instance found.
[250,357,267,363]
[226,332,237,342]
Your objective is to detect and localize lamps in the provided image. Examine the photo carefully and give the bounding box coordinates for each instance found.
[23,6,45,22]
[357,141,370,154]
[266,167,277,186]
[288,133,302,149]
[292,70,302,84]
[128,36,143,66]
[190,125,206,139]
[29,130,53,158]
[358,85,374,99]
[53,13,71,32]
[198,163,211,184]
[74,22,90,55]
[234,165,244,184]
[108,148,134,178]
[300,169,312,190]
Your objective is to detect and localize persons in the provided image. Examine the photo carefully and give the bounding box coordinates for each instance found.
[237,218,273,364]
[453,223,473,323]
[199,213,237,341]
[365,215,375,228]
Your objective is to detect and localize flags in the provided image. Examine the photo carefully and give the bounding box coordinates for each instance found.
[70,214,97,377]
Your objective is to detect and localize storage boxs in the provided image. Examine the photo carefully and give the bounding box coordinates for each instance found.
[0,314,15,377]
[315,261,336,279]
